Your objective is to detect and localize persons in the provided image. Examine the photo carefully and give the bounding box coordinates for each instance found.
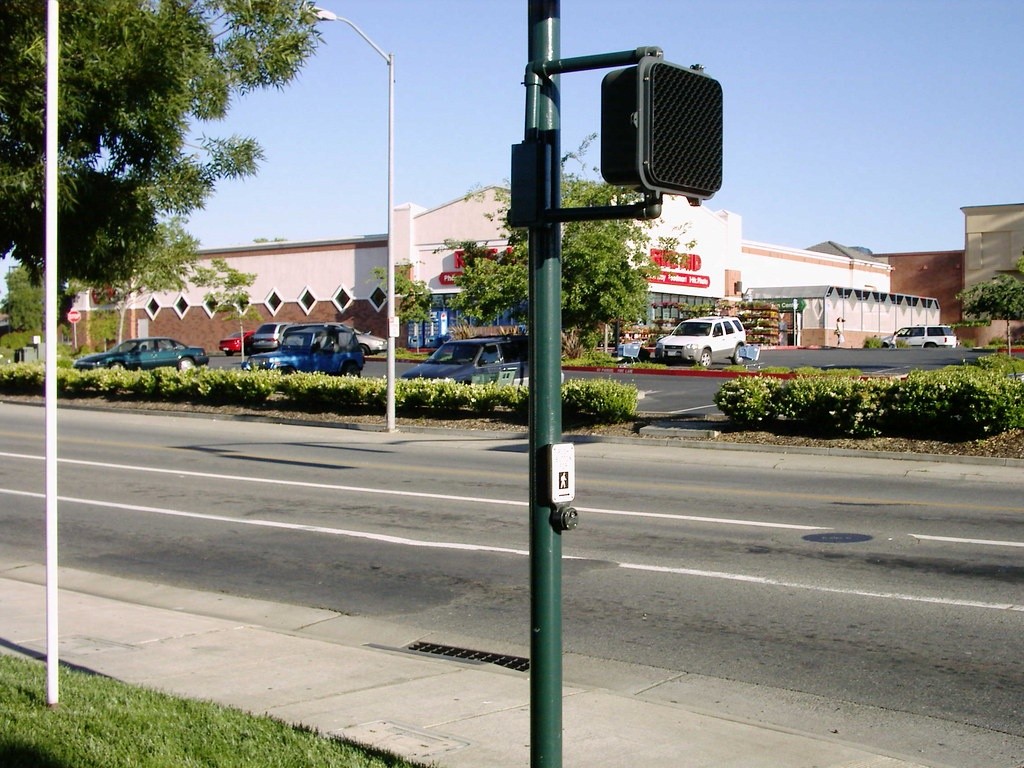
[888,331,897,349]
[836,317,843,345]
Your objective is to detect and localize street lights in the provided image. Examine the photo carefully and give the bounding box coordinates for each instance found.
[304,6,396,434]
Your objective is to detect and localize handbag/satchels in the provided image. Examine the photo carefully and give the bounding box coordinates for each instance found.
[834,330,840,335]
[838,334,844,343]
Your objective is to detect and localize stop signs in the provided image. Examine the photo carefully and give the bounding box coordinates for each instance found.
[67,312,81,323]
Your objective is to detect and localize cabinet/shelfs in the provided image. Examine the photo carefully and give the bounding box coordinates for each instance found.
[735,307,780,346]
[679,310,715,322]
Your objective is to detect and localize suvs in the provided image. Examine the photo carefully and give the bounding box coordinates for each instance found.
[880,326,957,348]
[242,327,364,377]
[400,334,565,388]
[655,316,747,366]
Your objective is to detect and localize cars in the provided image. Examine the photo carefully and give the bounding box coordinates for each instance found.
[73,336,210,371]
[219,322,388,356]
